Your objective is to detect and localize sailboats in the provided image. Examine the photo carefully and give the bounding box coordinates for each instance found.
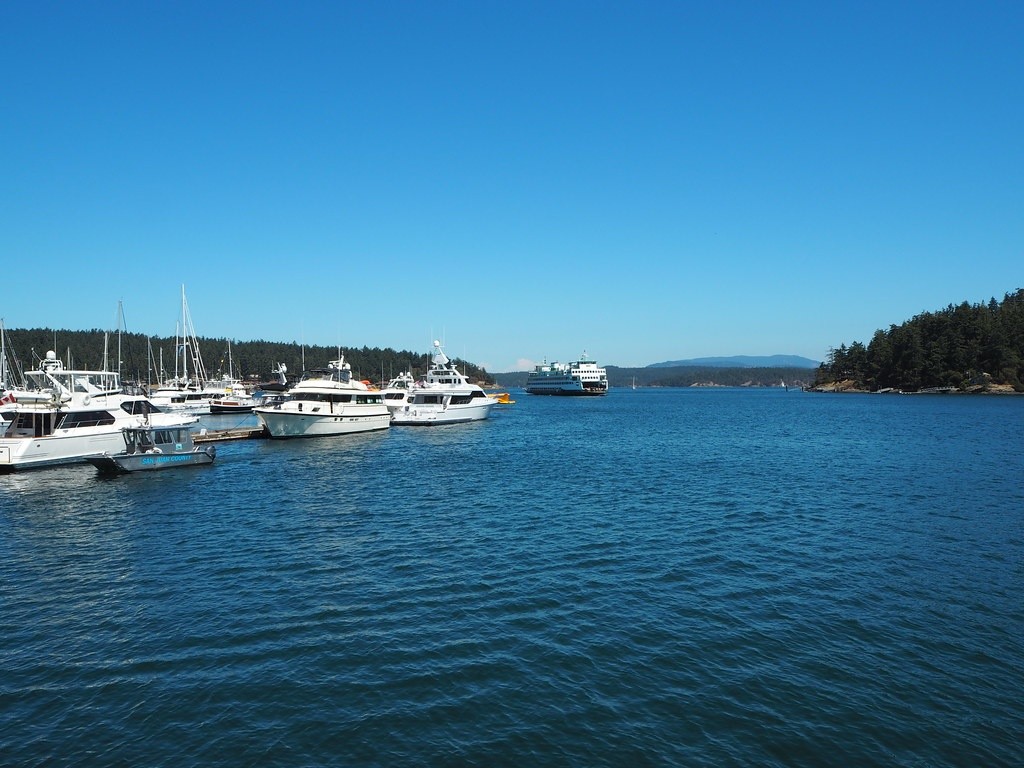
[0,282,414,471]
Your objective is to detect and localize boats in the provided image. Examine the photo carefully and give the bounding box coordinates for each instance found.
[251,340,391,439]
[525,349,609,396]
[379,325,500,425]
[84,409,216,475]
[487,392,516,405]
